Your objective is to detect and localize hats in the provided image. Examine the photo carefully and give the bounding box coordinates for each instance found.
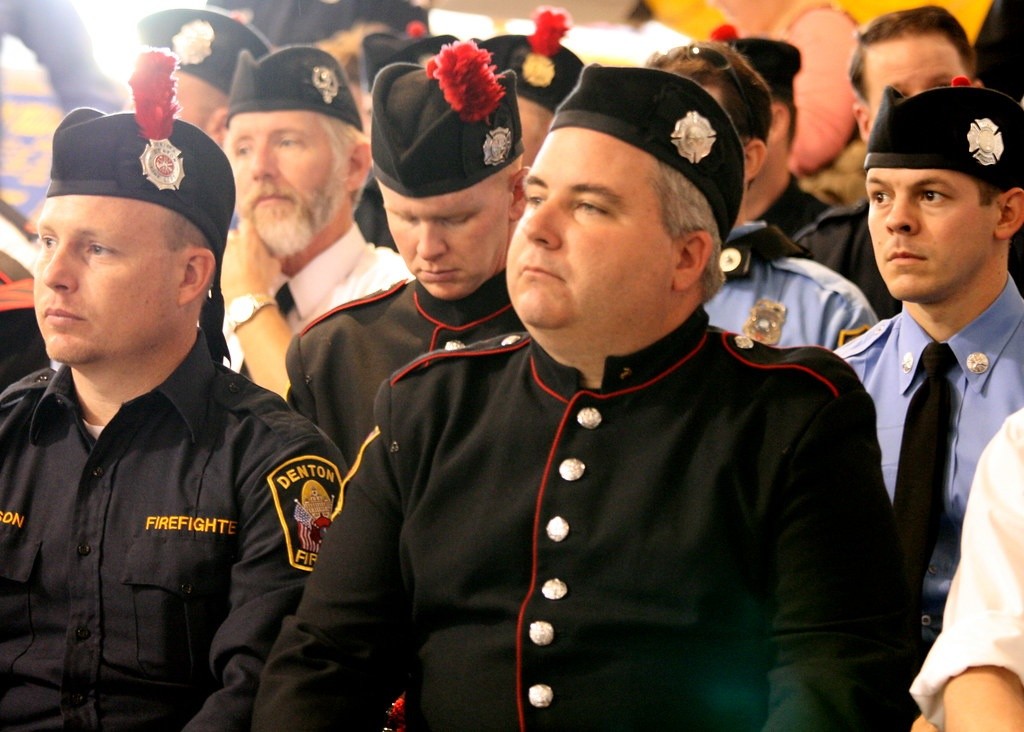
[476,5,584,114]
[549,62,745,252]
[226,46,364,132]
[711,25,800,102]
[864,75,1024,192]
[370,40,524,197]
[360,21,461,91]
[46,50,236,289]
[140,10,269,97]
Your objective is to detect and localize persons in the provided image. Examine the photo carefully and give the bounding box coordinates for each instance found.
[0,0,1024,732]
[250,64,922,732]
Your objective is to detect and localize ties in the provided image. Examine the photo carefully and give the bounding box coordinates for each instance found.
[238,283,295,382]
[891,343,958,667]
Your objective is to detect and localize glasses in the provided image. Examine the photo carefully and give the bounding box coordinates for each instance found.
[646,43,755,141]
